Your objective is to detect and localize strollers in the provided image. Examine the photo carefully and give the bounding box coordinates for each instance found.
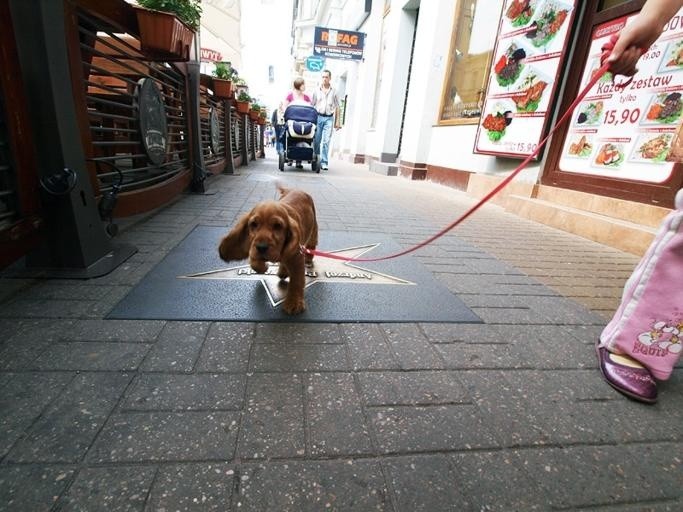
[273,104,320,173]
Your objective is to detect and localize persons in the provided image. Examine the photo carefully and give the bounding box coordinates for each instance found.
[270,70,340,170]
[595,0,683,404]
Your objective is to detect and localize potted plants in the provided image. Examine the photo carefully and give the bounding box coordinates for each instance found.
[133,0,267,125]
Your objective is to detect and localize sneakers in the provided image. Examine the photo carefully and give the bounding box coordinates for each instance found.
[321,163,329,170]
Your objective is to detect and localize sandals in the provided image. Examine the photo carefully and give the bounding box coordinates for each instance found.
[596,339,657,403]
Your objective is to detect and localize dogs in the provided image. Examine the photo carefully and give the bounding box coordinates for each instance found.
[219,183,319,316]
